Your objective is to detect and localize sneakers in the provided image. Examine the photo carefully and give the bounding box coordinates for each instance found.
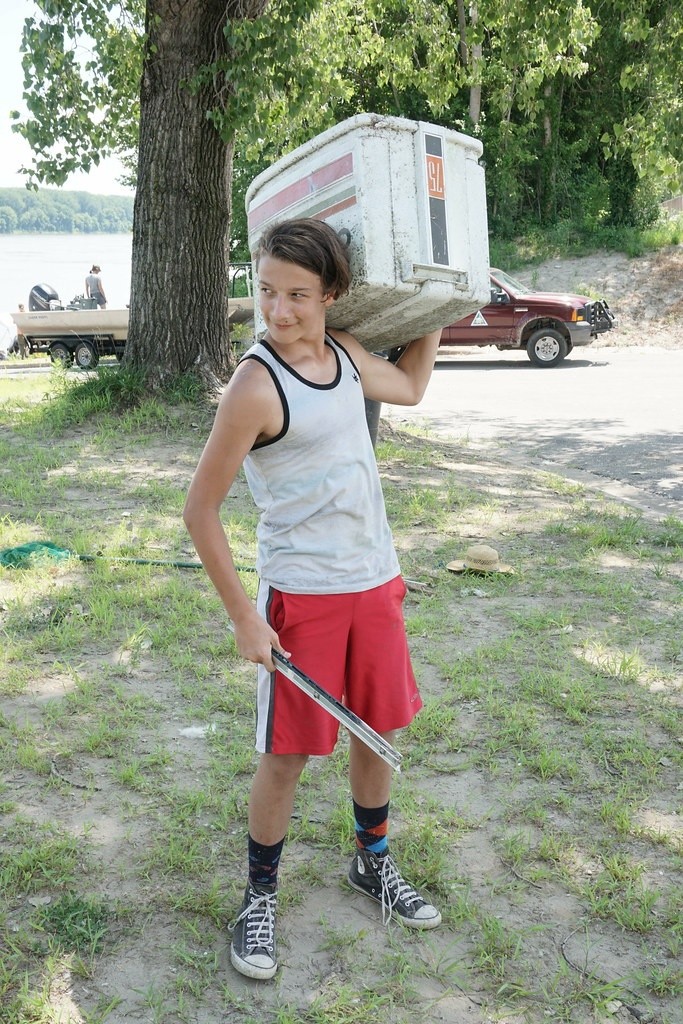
[348,842,442,930]
[227,878,278,980]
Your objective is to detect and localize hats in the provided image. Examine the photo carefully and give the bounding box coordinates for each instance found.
[92,265,101,271]
[446,544,511,573]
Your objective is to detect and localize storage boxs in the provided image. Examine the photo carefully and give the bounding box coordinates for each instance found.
[243,110,492,353]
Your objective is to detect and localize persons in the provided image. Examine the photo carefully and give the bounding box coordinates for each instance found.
[85,265,107,309]
[182,217,445,981]
[13,303,28,360]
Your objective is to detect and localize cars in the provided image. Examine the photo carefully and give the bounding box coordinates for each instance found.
[434,266,618,368]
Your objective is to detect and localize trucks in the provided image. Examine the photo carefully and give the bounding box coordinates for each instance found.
[10,257,255,370]
